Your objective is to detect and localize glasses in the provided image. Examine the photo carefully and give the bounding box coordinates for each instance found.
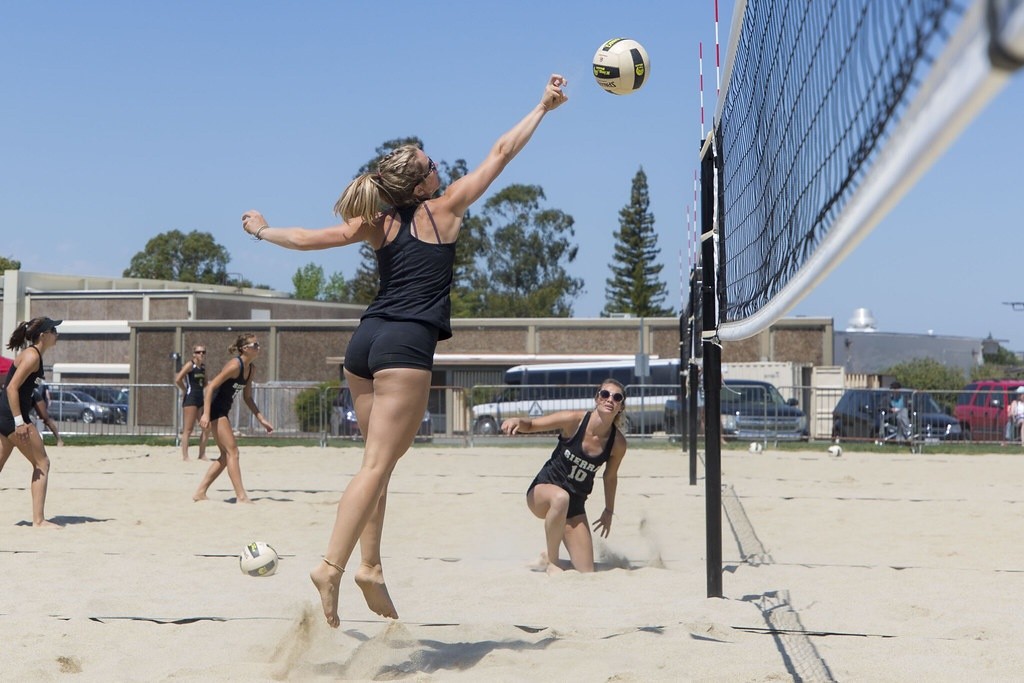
[239,343,260,350]
[43,328,57,335]
[598,390,624,403]
[411,157,436,193]
[193,351,207,355]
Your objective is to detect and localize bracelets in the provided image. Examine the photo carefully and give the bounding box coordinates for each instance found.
[255,225,270,241]
[14,415,23,426]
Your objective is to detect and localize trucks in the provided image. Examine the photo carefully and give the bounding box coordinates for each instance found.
[472,358,684,435]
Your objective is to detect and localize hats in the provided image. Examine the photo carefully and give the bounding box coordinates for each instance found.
[32,317,64,341]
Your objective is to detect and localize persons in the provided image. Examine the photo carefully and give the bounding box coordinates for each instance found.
[0,316,64,527]
[175,343,209,460]
[193,333,273,504]
[501,379,627,577]
[878,382,911,440]
[243,75,569,628]
[1010,386,1024,446]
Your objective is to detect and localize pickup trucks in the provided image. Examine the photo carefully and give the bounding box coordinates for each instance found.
[663,378,808,442]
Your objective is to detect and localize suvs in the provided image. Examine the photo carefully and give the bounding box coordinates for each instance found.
[951,379,1024,441]
[831,387,962,443]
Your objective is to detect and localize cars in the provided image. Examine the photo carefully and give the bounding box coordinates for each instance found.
[329,382,432,436]
[46,386,128,424]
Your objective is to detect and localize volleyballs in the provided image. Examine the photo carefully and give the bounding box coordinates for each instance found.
[750,442,762,453]
[592,37,650,95]
[828,445,842,457]
[239,541,280,577]
